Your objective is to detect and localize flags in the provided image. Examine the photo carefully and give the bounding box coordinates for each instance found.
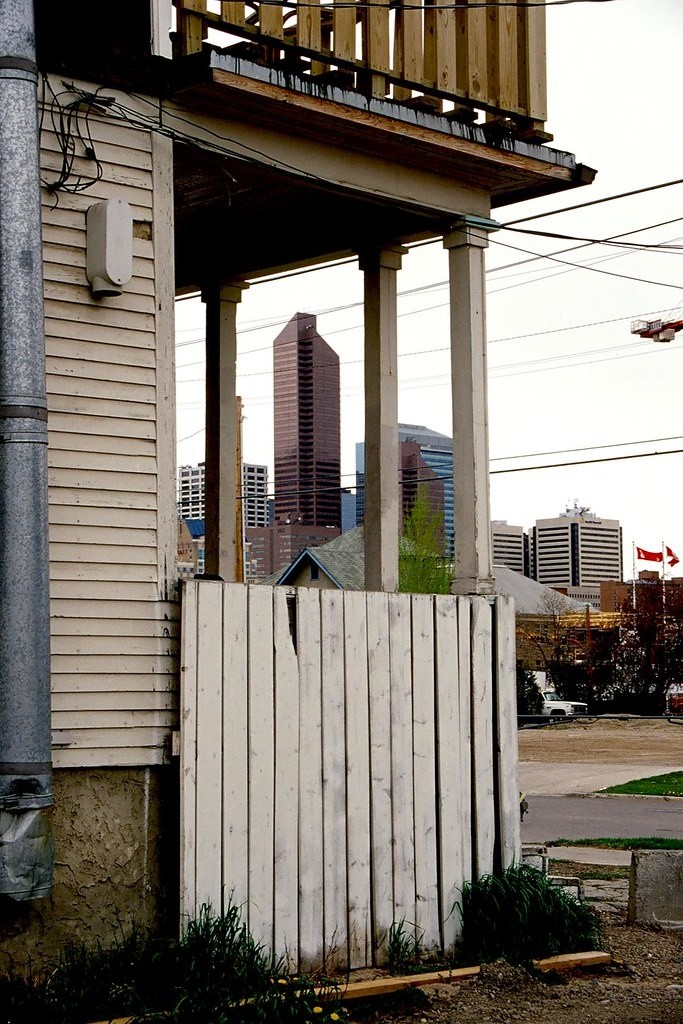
[636,547,663,562]
[666,546,680,567]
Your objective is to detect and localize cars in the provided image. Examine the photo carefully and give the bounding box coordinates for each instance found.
[538,690,589,724]
